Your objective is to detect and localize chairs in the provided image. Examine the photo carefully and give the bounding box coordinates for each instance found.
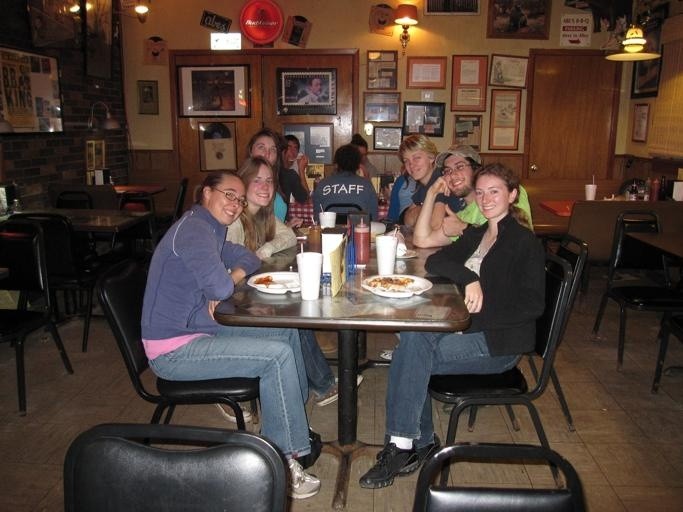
[529,198,683,394]
[444,232,589,430]
[428,253,572,486]
[96,248,259,431]
[62,424,291,511]
[1,175,192,415]
[412,443,586,511]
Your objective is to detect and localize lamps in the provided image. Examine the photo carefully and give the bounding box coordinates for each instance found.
[391,5,421,53]
[104,5,149,24]
[602,0,663,65]
[87,99,120,132]
[0,112,14,136]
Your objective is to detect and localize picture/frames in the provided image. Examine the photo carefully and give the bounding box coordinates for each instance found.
[630,102,651,146]
[135,79,158,116]
[628,1,668,100]
[366,50,398,90]
[453,114,482,152]
[281,123,334,166]
[363,91,401,123]
[406,56,447,89]
[488,89,522,150]
[487,54,530,88]
[482,0,554,43]
[196,121,238,173]
[451,55,488,113]
[403,100,445,138]
[177,64,251,117]
[373,126,404,150]
[276,68,337,116]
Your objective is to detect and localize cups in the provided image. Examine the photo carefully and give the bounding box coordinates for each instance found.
[318,210,336,228]
[295,252,321,301]
[583,184,596,201]
[376,236,395,275]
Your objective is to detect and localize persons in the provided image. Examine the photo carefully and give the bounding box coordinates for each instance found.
[3,66,31,113]
[299,79,326,103]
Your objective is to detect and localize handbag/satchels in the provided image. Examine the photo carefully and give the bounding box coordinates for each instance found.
[293,429,321,469]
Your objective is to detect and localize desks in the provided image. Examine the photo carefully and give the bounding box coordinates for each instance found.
[214,229,471,511]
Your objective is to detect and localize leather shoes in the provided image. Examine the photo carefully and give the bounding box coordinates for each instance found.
[443,401,485,416]
[314,332,338,353]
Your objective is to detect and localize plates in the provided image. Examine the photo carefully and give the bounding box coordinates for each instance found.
[396,249,419,259]
[246,271,302,294]
[362,274,433,297]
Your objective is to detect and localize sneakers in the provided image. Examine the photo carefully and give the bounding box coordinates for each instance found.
[214,403,253,423]
[360,445,420,490]
[286,455,320,499]
[312,374,364,407]
[378,348,395,360]
[401,433,441,477]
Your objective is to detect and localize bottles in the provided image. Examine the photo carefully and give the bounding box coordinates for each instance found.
[11,199,22,215]
[353,217,368,263]
[623,176,666,201]
[308,225,321,252]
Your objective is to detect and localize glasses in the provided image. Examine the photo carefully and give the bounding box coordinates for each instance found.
[441,163,472,177]
[212,186,248,208]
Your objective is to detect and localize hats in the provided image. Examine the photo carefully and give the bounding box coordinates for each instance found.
[435,144,482,168]
